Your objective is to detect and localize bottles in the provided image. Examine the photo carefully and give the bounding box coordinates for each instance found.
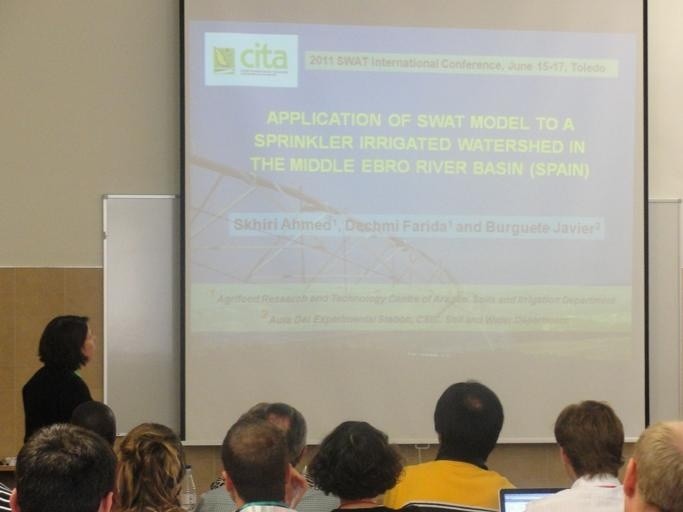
[179,465,198,511]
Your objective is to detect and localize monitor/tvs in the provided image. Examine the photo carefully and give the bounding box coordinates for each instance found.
[498,487,569,512]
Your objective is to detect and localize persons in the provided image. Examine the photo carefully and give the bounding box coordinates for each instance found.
[525,400,627,511]
[375,382,519,511]
[21,315,94,441]
[624,419,681,510]
[1,402,403,508]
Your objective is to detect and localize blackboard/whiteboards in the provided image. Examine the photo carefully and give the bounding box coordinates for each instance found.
[649,197,683,426]
[102,194,181,438]
